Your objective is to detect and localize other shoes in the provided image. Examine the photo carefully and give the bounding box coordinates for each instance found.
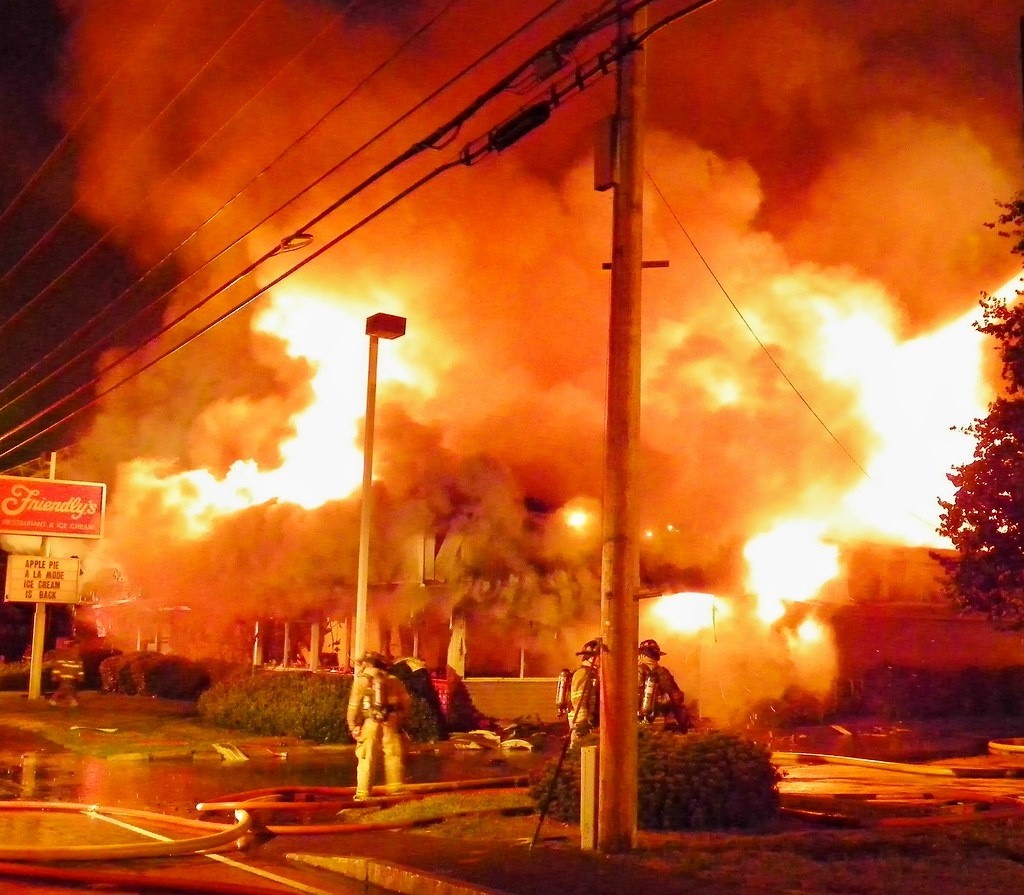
[49,699,57,707]
[353,795,368,801]
[70,700,79,707]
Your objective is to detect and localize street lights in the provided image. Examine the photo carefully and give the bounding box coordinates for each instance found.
[351,311,411,688]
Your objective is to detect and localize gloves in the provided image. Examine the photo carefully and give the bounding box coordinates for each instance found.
[575,723,592,736]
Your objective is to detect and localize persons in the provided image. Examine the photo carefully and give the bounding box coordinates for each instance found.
[346,657,412,802]
[48,641,84,712]
[637,639,685,734]
[567,639,600,748]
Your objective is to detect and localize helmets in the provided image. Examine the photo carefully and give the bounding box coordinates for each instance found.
[639,640,666,661]
[362,651,382,668]
[576,640,601,656]
